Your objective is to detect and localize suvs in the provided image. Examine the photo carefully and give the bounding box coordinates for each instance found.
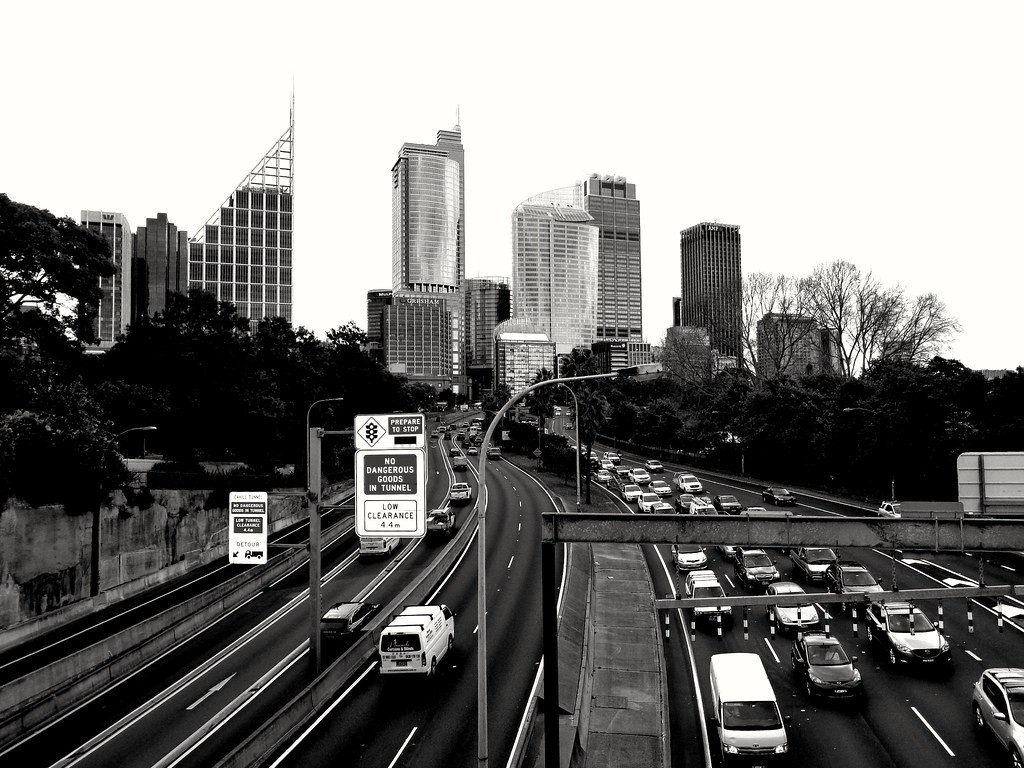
[735,547,781,590]
[824,560,886,616]
[877,500,902,518]
[972,667,1024,768]
[866,600,952,675]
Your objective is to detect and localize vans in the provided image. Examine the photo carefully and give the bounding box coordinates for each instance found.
[428,507,455,532]
[374,603,453,677]
[710,653,789,768]
[359,536,399,555]
[689,500,717,515]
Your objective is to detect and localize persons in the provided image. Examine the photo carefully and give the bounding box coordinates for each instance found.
[825,647,839,660]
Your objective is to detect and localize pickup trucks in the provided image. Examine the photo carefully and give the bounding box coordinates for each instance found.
[673,472,703,493]
[450,482,472,500]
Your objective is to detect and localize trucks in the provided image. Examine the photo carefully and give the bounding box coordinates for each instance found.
[789,548,839,583]
[245,550,264,559]
[684,570,732,627]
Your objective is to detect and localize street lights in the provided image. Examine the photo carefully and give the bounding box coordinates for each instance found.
[306,398,345,491]
[557,382,581,512]
[477,359,663,768]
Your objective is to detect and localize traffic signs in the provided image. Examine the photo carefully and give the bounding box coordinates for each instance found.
[229,491,268,565]
[353,414,428,538]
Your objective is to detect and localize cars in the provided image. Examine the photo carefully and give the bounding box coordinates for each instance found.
[649,502,678,514]
[628,468,651,484]
[762,487,796,505]
[648,481,673,495]
[718,546,744,559]
[638,493,663,512]
[790,631,863,704]
[745,507,766,512]
[430,401,632,489]
[319,600,381,644]
[763,581,819,630]
[622,485,644,501]
[644,459,662,472]
[677,494,696,510]
[672,544,708,571]
[714,495,742,513]
